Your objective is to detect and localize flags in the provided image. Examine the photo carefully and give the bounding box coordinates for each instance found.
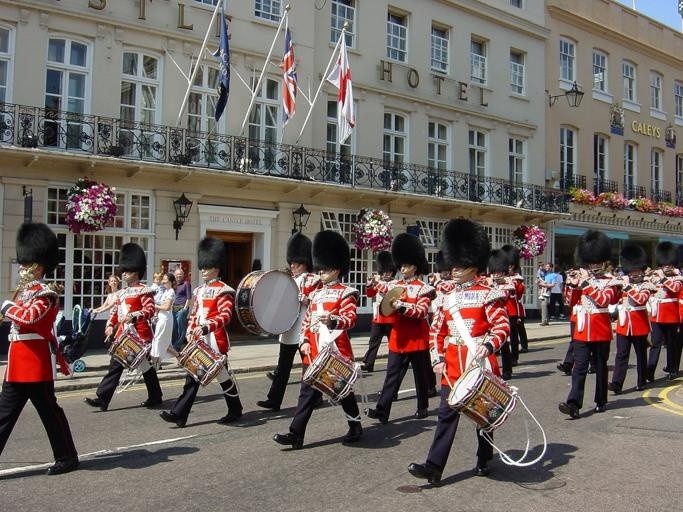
[212,4,230,123]
[326,33,356,147]
[282,16,298,129]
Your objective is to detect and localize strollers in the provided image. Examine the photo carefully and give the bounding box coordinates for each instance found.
[49,304,97,372]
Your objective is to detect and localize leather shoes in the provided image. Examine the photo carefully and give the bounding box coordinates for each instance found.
[556,363,572,376]
[594,402,609,413]
[376,391,399,402]
[47,456,80,475]
[634,384,647,390]
[343,423,364,444]
[360,364,375,373]
[608,382,623,394]
[140,396,163,408]
[364,408,389,424]
[257,400,282,411]
[559,402,580,419]
[219,411,243,424]
[266,371,277,380]
[159,410,187,427]
[587,367,597,374]
[407,462,443,485]
[474,462,492,477]
[415,408,430,418]
[83,396,109,412]
[426,388,438,399]
[273,433,304,449]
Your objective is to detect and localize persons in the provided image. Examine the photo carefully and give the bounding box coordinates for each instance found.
[557,266,598,376]
[272,230,363,450]
[538,263,558,326]
[536,261,550,300]
[159,236,243,428]
[83,243,163,412]
[500,244,528,353]
[550,264,567,321]
[487,249,526,366]
[407,217,512,486]
[645,242,683,382]
[150,272,167,321]
[172,265,192,351]
[150,272,186,371]
[435,250,453,287]
[427,273,438,288]
[608,267,622,333]
[603,259,615,279]
[558,230,626,419]
[93,274,123,316]
[360,252,397,373]
[0,221,81,476]
[479,276,516,381]
[363,233,437,424]
[599,244,659,393]
[256,231,325,411]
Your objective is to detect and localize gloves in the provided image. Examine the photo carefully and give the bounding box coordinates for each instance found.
[1,299,16,310]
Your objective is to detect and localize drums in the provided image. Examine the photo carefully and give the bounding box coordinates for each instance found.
[176,340,229,387]
[447,364,521,431]
[301,347,360,402]
[235,271,302,337]
[110,329,149,369]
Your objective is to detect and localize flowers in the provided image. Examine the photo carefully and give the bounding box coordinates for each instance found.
[513,221,547,260]
[572,186,682,219]
[352,204,393,252]
[65,175,119,235]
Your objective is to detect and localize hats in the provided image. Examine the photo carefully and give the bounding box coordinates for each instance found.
[573,247,585,267]
[488,249,509,274]
[655,241,676,264]
[415,257,429,277]
[502,245,520,268]
[198,238,227,277]
[437,250,453,273]
[118,243,147,279]
[16,222,58,274]
[441,218,490,273]
[376,251,397,275]
[286,234,313,273]
[578,230,611,263]
[675,244,683,269]
[621,244,646,273]
[313,230,351,277]
[393,233,425,268]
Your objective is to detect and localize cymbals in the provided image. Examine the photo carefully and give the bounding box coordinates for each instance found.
[378,287,409,318]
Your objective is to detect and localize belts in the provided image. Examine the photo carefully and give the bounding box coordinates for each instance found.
[449,336,485,345]
[577,308,610,314]
[8,333,44,342]
[629,306,647,311]
[661,297,678,303]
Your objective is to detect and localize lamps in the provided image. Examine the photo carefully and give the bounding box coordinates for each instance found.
[172,193,192,240]
[291,203,312,235]
[546,80,585,107]
